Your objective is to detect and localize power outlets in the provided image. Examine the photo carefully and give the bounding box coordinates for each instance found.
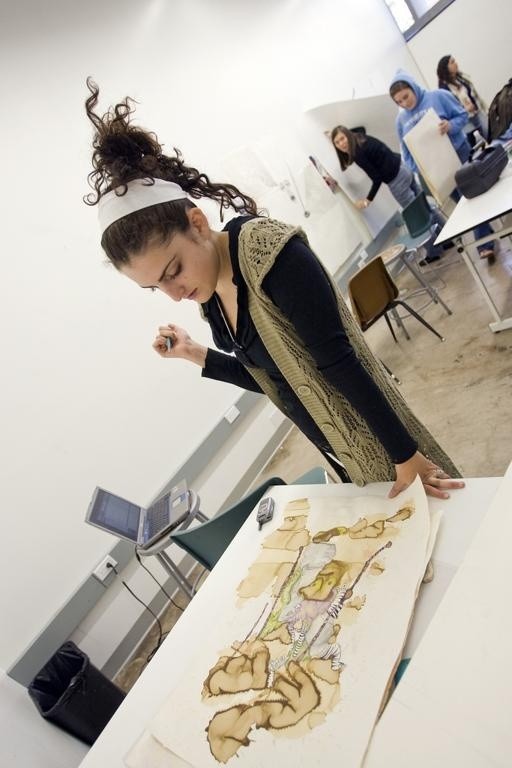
[92,555,118,584]
[224,405,240,424]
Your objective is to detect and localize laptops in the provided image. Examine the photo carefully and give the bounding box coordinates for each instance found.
[84,478,190,548]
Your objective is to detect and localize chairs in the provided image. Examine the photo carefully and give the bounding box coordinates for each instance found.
[349,256,446,386]
[169,465,337,572]
[393,191,448,287]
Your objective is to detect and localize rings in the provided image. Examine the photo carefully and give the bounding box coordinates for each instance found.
[437,469,444,477]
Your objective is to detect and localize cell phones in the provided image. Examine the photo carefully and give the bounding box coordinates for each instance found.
[256,497,275,532]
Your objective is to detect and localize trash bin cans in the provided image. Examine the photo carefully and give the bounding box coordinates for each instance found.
[27,641,126,748]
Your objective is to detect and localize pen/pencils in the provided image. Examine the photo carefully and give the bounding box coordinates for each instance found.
[167,336,171,352]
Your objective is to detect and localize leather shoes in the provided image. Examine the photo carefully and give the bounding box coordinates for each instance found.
[441,242,454,250]
[419,253,440,267]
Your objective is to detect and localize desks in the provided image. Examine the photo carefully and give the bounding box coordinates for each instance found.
[79,477,512,767]
[433,157,512,333]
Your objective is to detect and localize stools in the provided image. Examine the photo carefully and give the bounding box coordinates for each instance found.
[137,488,210,601]
[363,245,452,342]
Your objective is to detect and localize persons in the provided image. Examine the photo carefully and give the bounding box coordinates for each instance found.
[82,78,465,500]
[324,55,499,266]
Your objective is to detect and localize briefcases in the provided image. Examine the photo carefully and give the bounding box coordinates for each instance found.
[454,139,509,198]
